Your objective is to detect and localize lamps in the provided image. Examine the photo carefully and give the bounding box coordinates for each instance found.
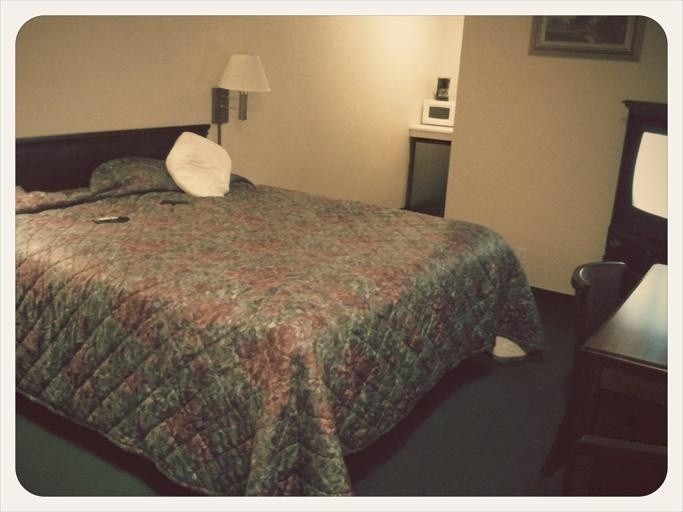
[212,50,272,123]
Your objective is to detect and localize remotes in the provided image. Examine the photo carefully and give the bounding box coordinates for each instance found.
[92,216,129,224]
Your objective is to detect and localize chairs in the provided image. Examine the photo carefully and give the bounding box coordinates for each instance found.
[539,261,667,497]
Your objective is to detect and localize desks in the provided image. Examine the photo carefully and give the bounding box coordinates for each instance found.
[405,123,453,210]
[563,261,667,497]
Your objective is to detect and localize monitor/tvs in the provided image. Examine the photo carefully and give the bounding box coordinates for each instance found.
[622,124,668,243]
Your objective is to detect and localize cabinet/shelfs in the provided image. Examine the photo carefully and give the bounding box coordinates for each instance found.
[601,225,667,292]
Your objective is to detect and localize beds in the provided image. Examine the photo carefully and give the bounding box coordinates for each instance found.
[16,124,549,496]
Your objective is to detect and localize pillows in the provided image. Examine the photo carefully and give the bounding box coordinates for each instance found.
[90,155,246,199]
[165,131,232,197]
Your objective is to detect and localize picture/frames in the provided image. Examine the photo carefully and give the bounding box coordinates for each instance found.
[528,15,646,62]
